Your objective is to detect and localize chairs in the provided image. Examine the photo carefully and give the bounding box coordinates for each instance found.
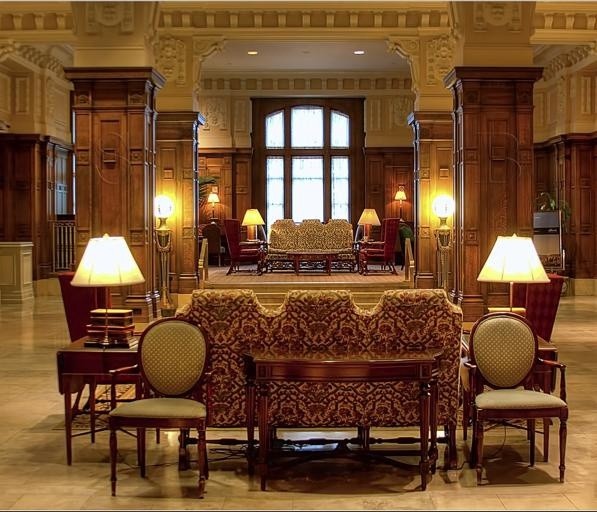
[202,224,225,267]
[57,272,136,418]
[462,312,568,486]
[224,219,268,276]
[107,319,210,498]
[498,274,564,343]
[359,218,400,276]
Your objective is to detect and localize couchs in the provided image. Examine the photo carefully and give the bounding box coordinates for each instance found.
[176,289,464,471]
[265,219,358,272]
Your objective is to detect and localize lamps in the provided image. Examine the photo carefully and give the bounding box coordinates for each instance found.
[357,208,382,241]
[153,195,176,315]
[433,196,456,289]
[70,233,146,346]
[242,208,265,241]
[207,194,220,218]
[395,191,406,217]
[476,233,551,312]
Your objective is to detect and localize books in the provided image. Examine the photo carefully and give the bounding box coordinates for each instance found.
[84,308,138,348]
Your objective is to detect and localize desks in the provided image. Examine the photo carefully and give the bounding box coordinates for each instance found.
[242,350,444,491]
[465,333,558,462]
[57,334,142,466]
